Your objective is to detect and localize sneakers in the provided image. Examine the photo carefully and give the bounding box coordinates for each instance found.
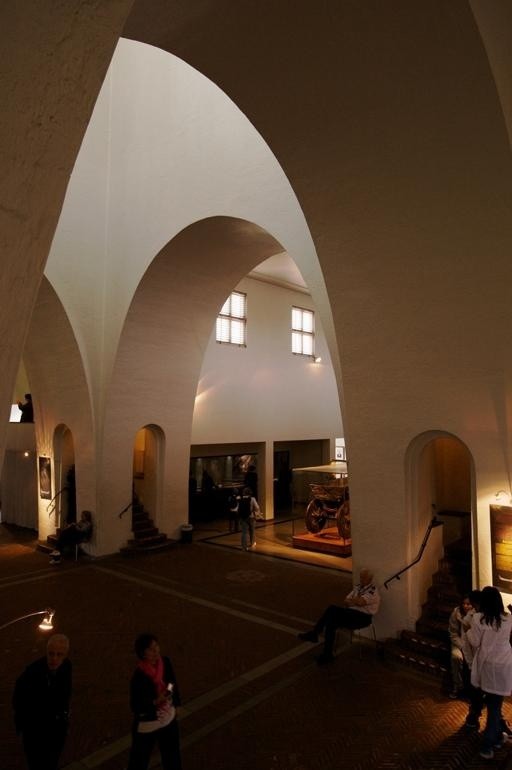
[49,558,61,564]
[449,688,458,697]
[49,551,61,556]
[244,543,256,551]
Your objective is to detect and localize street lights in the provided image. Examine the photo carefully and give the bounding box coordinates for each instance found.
[1,604,57,634]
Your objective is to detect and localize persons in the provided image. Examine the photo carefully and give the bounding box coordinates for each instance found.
[66,462,77,524]
[295,567,380,665]
[48,510,95,565]
[244,465,259,502]
[445,585,512,762]
[128,630,184,770]
[188,470,201,529]
[11,632,77,769]
[237,487,261,552]
[227,487,242,533]
[16,393,34,423]
[200,470,219,524]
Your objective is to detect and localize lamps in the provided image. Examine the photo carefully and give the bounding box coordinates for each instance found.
[0,608,55,633]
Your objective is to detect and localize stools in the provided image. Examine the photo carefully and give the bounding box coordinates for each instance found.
[76,539,91,561]
[348,622,377,647]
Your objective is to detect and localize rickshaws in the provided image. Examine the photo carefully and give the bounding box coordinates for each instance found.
[304,477,350,540]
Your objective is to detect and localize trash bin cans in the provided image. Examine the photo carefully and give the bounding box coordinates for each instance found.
[180,524,193,544]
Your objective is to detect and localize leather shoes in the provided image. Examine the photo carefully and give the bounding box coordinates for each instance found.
[298,632,318,643]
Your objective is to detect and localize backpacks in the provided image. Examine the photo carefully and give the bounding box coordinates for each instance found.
[229,495,237,508]
[238,496,251,518]
